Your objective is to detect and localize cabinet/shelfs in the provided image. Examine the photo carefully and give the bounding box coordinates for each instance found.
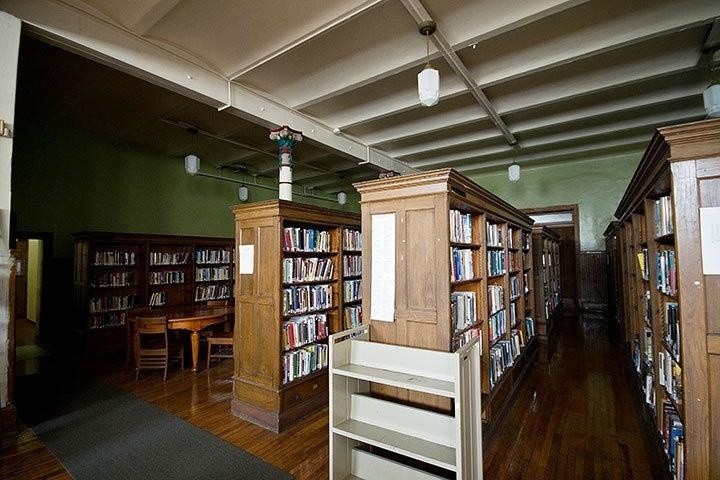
[230,200,362,434]
[605,117,719,480]
[352,169,536,433]
[668,154,719,479]
[536,224,562,341]
[68,229,236,359]
[329,323,483,480]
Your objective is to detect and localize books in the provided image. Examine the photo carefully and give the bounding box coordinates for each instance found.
[449,208,561,392]
[281,228,364,384]
[627,192,685,480]
[88,247,236,328]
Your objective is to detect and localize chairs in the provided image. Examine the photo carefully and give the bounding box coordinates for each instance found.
[126,300,236,382]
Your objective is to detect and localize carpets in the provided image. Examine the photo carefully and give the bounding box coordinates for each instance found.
[12,353,295,480]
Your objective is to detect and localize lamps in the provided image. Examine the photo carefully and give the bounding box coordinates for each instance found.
[238,167,248,201]
[184,127,201,177]
[335,175,347,205]
[508,142,520,183]
[701,42,720,118]
[417,21,441,108]
